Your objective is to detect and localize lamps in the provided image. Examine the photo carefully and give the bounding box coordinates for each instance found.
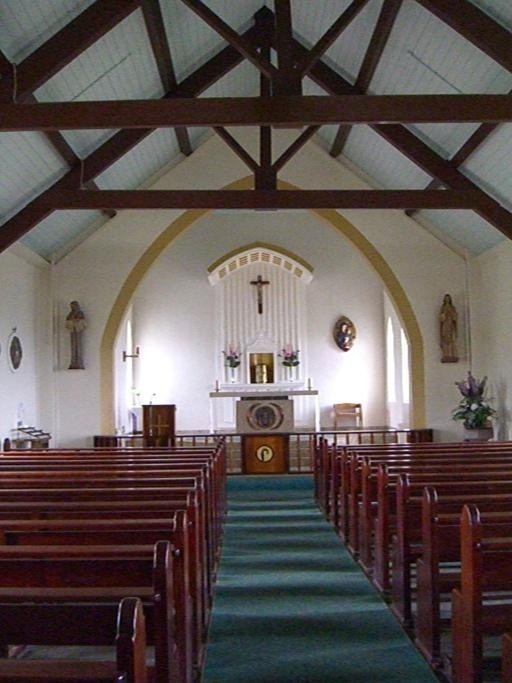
[123,346,140,361]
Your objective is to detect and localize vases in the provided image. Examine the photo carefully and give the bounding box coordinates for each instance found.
[462,421,494,440]
[290,367,296,382]
[230,368,237,380]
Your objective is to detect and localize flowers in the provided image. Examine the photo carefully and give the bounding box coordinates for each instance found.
[278,346,301,366]
[451,372,496,429]
[221,348,241,367]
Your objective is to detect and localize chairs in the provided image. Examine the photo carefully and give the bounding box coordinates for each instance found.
[334,403,362,430]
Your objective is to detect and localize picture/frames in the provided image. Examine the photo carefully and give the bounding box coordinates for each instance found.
[8,334,24,373]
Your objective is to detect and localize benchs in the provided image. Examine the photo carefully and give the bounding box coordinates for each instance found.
[0,435,227,683]
[313,434,512,683]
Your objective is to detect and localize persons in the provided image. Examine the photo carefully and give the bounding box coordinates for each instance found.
[65,301,86,367]
[439,293,457,356]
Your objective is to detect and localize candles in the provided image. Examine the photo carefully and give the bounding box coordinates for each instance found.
[216,379,220,389]
[308,377,312,387]
